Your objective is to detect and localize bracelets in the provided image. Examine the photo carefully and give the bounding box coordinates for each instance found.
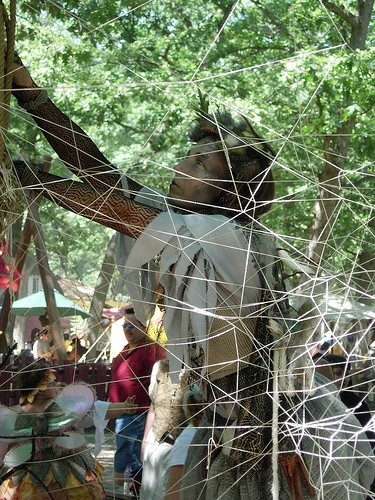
[19,162,40,186]
[23,87,46,112]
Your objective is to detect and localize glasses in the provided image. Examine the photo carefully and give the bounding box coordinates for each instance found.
[121,322,145,330]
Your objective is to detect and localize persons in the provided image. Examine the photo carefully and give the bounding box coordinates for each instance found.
[4,43,317,500]
[4,364,137,433]
[108,306,168,490]
[27,324,91,364]
[322,353,375,457]
